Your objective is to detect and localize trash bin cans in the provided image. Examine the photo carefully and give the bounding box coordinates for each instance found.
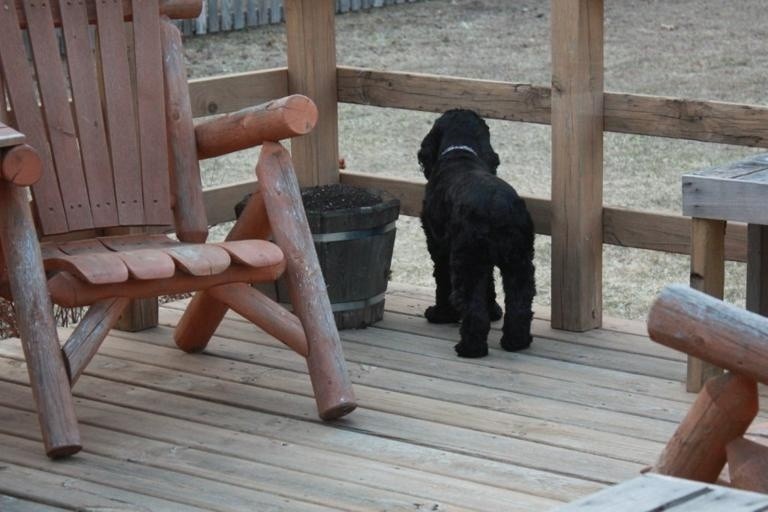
[233,184,400,330]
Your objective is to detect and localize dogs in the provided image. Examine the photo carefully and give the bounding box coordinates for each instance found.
[418,109,535,357]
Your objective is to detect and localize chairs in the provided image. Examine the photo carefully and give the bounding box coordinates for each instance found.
[563,288,765,510]
[0,0,359,458]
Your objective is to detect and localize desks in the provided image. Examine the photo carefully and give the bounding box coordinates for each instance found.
[681,151,766,392]
